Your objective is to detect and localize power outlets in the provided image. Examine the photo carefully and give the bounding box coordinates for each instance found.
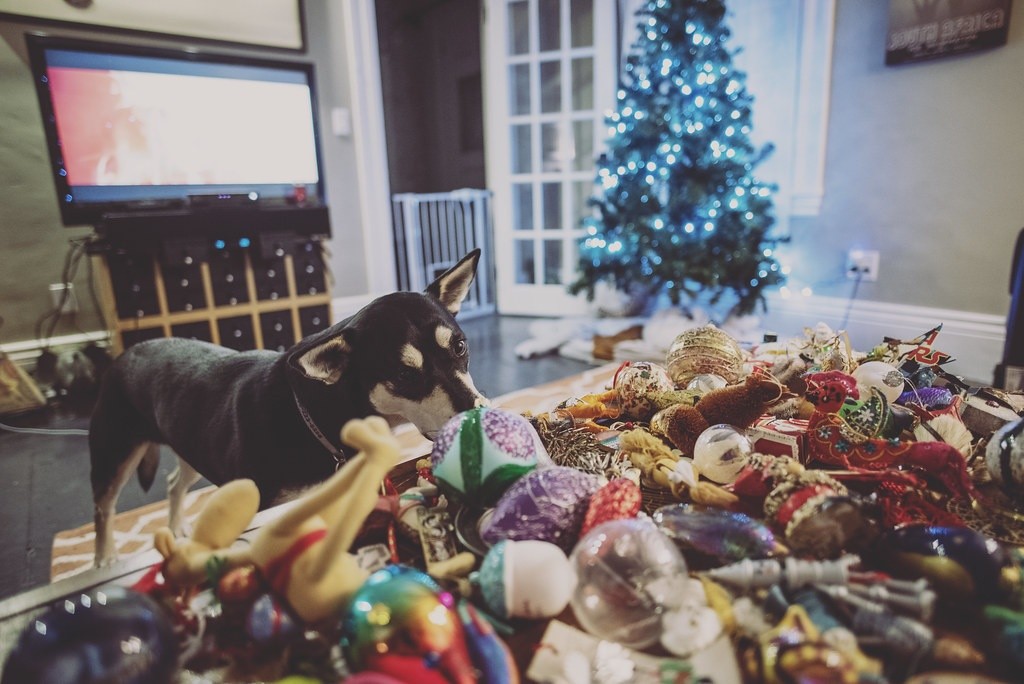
[846,250,880,283]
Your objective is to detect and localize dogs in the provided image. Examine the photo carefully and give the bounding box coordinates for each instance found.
[79,247,489,567]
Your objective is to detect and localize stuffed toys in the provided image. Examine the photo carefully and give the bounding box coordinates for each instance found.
[658,372,780,459]
[151,415,408,628]
[804,370,992,507]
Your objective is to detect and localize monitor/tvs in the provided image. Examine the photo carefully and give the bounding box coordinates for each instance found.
[24,30,323,227]
